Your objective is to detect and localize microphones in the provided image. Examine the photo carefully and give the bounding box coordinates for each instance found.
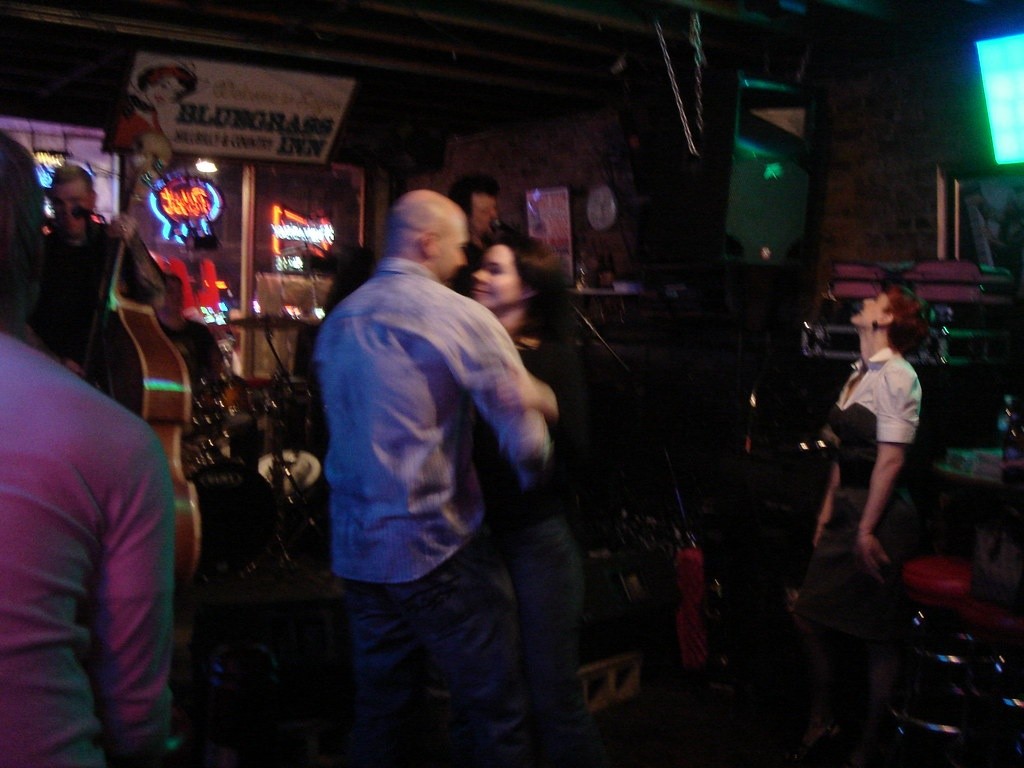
[70,205,96,219]
[492,219,524,242]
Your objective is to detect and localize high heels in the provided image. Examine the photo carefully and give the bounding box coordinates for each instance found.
[783,721,844,766]
[840,745,884,768]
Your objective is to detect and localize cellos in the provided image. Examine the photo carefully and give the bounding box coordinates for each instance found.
[83,129,204,596]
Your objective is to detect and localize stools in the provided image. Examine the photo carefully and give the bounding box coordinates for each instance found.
[884,553,1024,768]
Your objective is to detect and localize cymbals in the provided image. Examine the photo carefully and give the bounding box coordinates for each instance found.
[228,313,304,330]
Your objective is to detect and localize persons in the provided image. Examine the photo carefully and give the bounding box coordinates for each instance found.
[781,285,922,767]
[1,126,173,768]
[449,172,502,305]
[23,161,166,375]
[314,188,607,768]
[156,274,224,394]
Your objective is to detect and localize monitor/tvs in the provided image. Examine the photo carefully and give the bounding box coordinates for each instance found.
[974,30,1024,171]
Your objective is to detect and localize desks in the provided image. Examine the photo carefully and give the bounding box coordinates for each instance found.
[567,286,648,297]
[193,560,343,768]
[933,447,1024,493]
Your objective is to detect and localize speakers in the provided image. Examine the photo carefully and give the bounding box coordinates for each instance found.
[719,79,807,269]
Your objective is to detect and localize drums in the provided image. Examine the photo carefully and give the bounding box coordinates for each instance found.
[257,447,323,494]
[221,375,255,431]
